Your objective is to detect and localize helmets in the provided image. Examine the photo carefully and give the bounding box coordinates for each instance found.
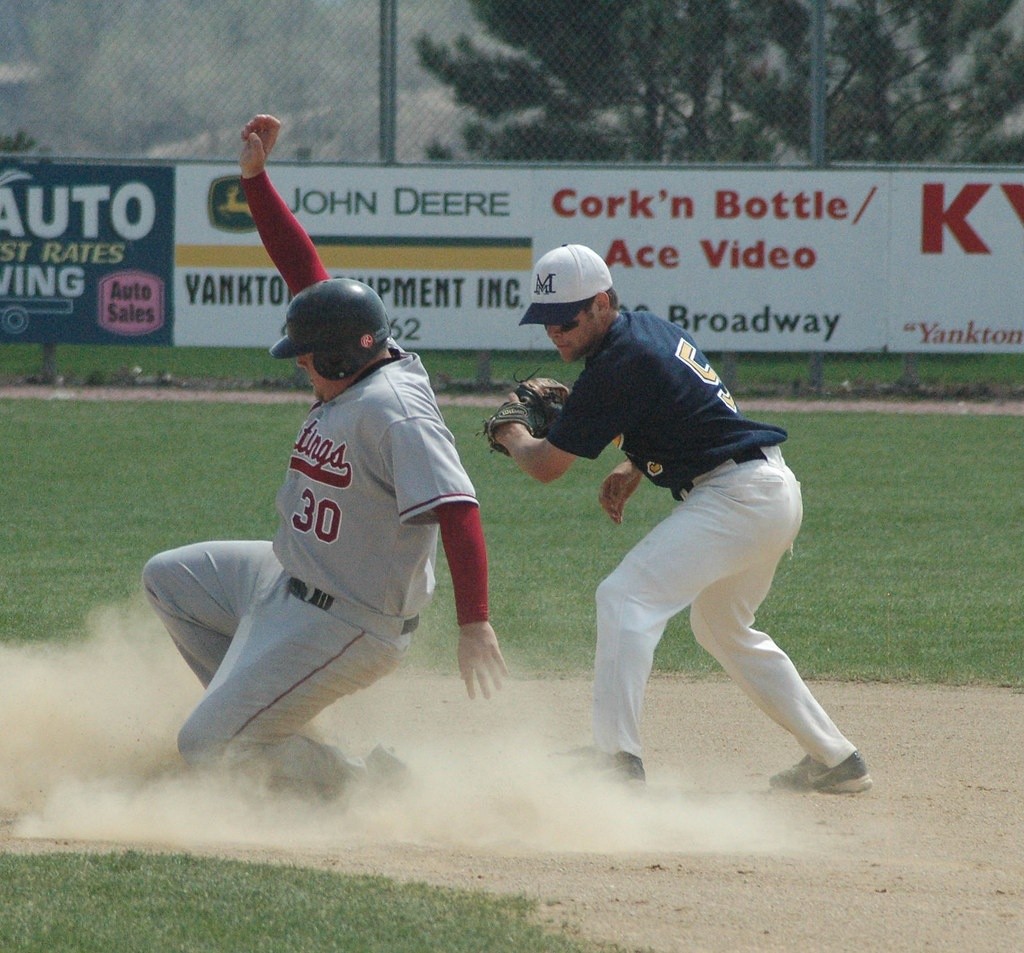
[267,278,392,380]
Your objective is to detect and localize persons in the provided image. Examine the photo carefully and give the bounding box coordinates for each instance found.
[140,112,510,804]
[476,241,875,794]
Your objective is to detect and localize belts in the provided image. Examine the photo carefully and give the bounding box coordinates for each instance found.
[683,447,767,493]
[285,578,421,638]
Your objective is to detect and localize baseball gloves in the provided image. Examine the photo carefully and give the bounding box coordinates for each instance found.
[478,377,570,455]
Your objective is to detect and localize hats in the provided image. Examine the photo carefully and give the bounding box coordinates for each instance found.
[518,243,613,328]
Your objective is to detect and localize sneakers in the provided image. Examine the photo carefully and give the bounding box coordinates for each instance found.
[595,754,649,796]
[770,749,872,794]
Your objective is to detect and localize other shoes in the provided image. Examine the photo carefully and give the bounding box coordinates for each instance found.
[331,753,382,813]
